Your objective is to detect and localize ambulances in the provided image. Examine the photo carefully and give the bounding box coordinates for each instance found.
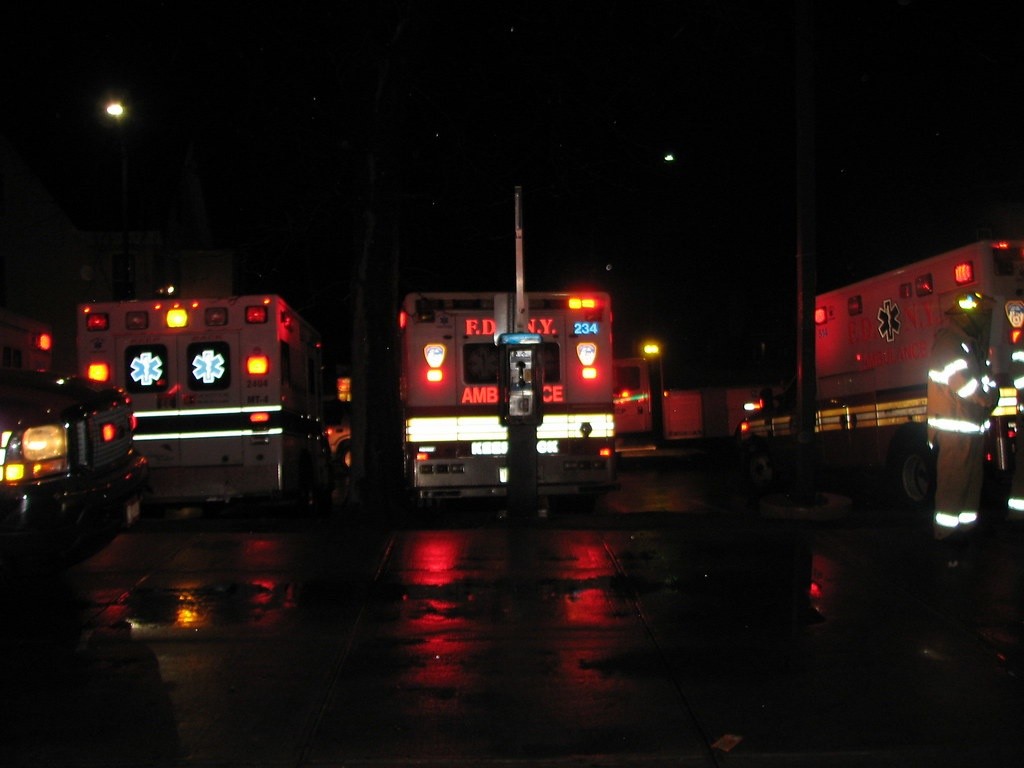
[812,238,1024,509]
[78,294,332,529]
[398,289,617,522]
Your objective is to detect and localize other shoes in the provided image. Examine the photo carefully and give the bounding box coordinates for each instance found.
[966,529,998,560]
[942,532,972,564]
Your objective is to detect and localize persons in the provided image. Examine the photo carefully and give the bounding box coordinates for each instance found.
[926,293,1003,566]
[1006,332,1024,519]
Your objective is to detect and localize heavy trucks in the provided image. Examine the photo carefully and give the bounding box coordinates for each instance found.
[613,356,788,479]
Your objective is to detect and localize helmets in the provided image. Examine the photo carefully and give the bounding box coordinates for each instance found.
[945,291,998,315]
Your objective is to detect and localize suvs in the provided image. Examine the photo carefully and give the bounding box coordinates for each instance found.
[0,367,148,591]
